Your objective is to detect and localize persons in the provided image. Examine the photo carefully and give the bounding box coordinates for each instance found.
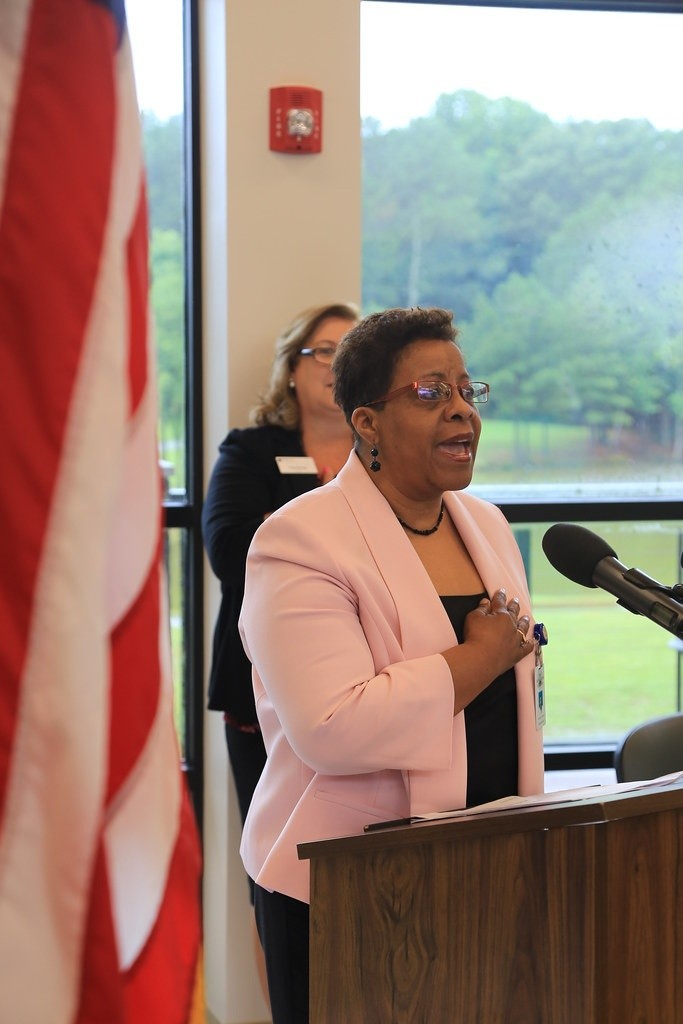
[205,304,548,1024]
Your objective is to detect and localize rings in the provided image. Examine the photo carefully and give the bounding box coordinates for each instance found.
[516,629,529,647]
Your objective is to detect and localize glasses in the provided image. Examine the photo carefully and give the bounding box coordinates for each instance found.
[301,348,338,364]
[364,380,490,405]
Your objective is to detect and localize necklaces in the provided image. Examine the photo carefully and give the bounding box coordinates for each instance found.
[392,500,447,537]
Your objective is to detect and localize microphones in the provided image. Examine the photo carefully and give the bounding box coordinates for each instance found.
[541,524,683,640]
